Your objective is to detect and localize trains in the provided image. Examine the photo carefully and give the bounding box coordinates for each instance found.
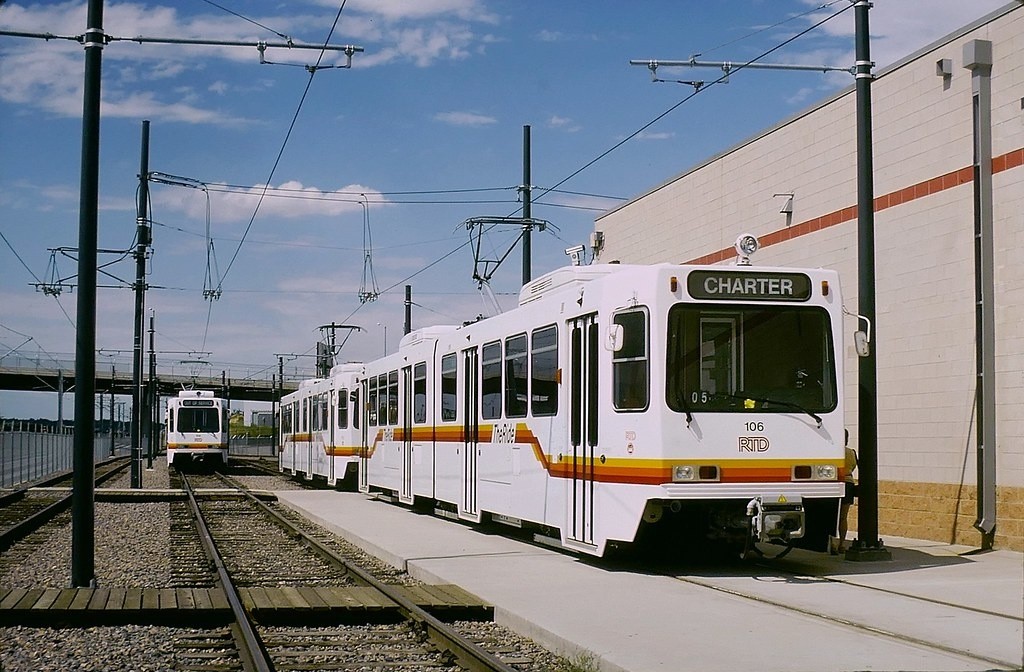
[164,390,229,468]
[274,260,872,566]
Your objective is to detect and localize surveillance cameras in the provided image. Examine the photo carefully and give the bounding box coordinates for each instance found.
[564,245,585,255]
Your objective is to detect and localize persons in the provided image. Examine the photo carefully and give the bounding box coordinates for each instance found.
[838,429,858,552]
